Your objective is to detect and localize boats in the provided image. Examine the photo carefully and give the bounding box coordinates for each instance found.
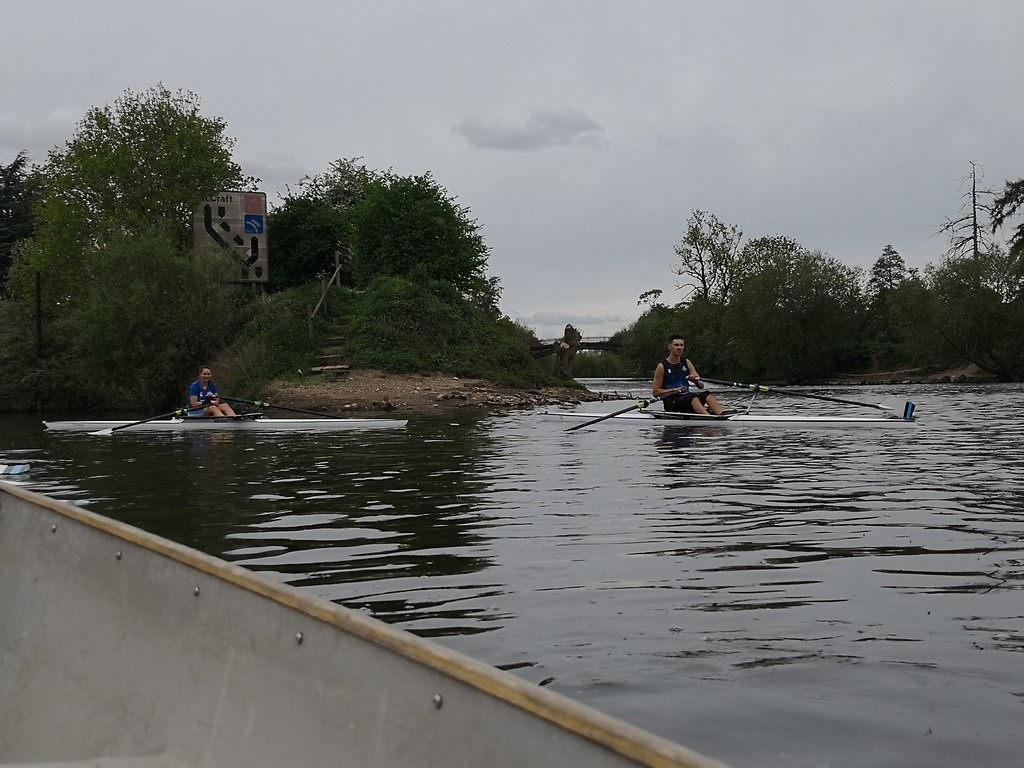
[40,419,410,433]
[537,408,915,422]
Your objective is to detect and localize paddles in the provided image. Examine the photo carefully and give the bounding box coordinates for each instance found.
[563,389,687,432]
[685,374,895,411]
[86,403,215,437]
[209,394,350,420]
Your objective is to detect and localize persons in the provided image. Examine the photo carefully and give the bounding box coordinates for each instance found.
[653,335,722,415]
[188,365,236,416]
